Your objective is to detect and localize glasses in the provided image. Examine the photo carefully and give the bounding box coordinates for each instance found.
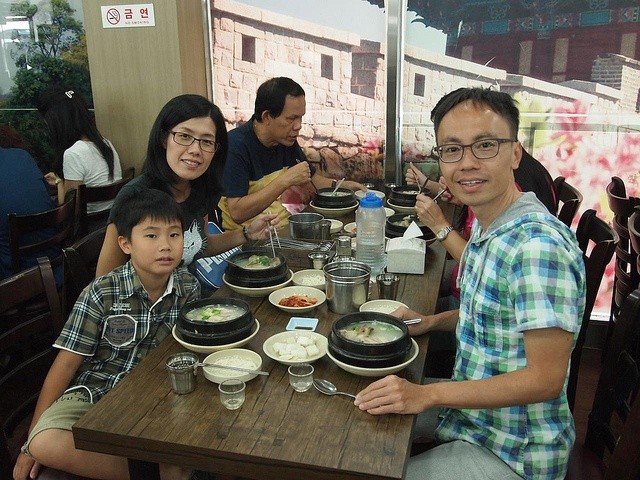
[434,138,517,163]
[168,129,220,153]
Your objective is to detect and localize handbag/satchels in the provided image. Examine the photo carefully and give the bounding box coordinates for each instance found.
[190,220,242,298]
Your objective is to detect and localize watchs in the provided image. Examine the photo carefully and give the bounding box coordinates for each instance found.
[55,177,62,189]
[435,224,454,242]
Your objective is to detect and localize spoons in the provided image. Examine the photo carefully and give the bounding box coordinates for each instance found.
[264,238,332,252]
[312,379,356,400]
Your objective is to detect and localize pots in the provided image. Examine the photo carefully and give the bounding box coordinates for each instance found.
[225,252,289,288]
[386,213,436,240]
[315,187,356,208]
[328,312,412,367]
[175,297,255,346]
[388,186,431,207]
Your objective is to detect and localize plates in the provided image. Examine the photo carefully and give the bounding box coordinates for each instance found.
[285,317,319,331]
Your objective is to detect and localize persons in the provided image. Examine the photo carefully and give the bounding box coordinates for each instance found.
[405,139,558,312]
[96,94,280,300]
[354,85,588,479]
[13,189,202,479]
[218,77,368,245]
[1,146,63,288]
[42,98,122,233]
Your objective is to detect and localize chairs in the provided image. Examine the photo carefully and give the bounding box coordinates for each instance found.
[567,208,620,413]
[600,175,640,368]
[0,255,91,480]
[77,167,135,238]
[553,174,583,228]
[7,188,78,313]
[567,293,638,479]
[62,226,107,294]
[627,205,640,290]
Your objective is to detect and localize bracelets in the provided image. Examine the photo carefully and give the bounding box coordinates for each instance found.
[242,225,250,243]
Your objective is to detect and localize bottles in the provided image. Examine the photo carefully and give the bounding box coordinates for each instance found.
[356,192,386,275]
[335,236,352,259]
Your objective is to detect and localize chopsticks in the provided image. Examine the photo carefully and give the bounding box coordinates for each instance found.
[266,212,282,258]
[404,319,423,326]
[196,361,270,377]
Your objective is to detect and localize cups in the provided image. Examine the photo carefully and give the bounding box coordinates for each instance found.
[307,252,330,269]
[218,379,246,410]
[376,274,401,300]
[287,363,315,393]
[165,352,199,394]
[322,220,332,240]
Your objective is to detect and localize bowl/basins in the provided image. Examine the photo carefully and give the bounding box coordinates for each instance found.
[322,218,344,233]
[385,236,438,248]
[171,319,261,354]
[268,286,327,313]
[201,347,263,386]
[262,330,329,365]
[359,298,409,319]
[309,201,360,219]
[344,222,356,235]
[325,337,421,378]
[222,269,294,298]
[386,199,419,215]
[293,269,326,290]
[355,189,386,206]
[385,207,395,223]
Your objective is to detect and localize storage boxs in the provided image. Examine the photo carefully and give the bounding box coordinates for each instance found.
[386,237,426,275]
